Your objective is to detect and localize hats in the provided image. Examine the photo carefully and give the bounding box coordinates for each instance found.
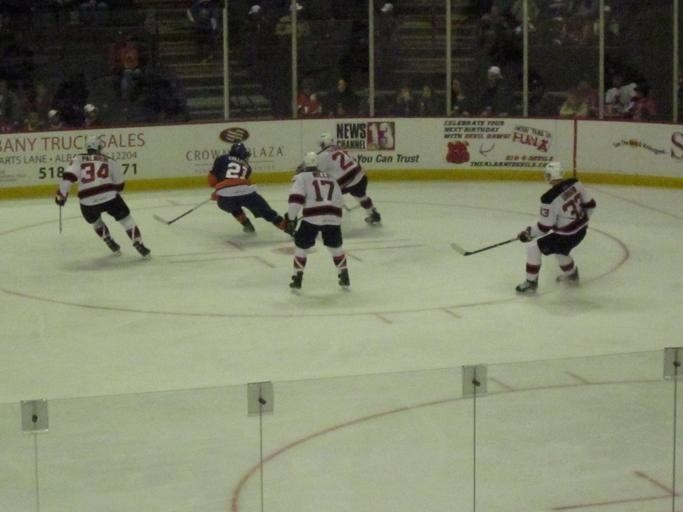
[47,110,58,118]
[83,105,95,112]
[488,66,504,80]
[289,4,302,12]
[381,4,395,12]
[248,5,262,16]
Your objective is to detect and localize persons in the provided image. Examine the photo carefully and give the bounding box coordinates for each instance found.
[516,162,596,294]
[208,142,297,237]
[55,138,149,258]
[286,152,354,293]
[238,1,442,119]
[187,2,217,62]
[450,1,675,122]
[2,2,187,130]
[317,132,380,224]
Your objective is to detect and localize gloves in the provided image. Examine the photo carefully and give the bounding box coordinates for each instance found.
[517,227,532,242]
[285,221,295,233]
[55,195,67,206]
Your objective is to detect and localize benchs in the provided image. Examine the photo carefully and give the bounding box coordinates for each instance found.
[0,0,683,123]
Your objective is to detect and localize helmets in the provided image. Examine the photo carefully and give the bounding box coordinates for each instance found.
[86,136,102,152]
[229,144,246,157]
[304,151,318,168]
[545,162,563,184]
[319,132,333,148]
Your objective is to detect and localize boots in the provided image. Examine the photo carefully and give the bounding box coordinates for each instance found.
[132,241,150,256]
[284,226,297,237]
[289,271,302,288]
[103,236,119,252]
[557,267,578,282]
[364,208,379,224]
[337,268,349,286]
[516,276,537,292]
[241,218,255,233]
